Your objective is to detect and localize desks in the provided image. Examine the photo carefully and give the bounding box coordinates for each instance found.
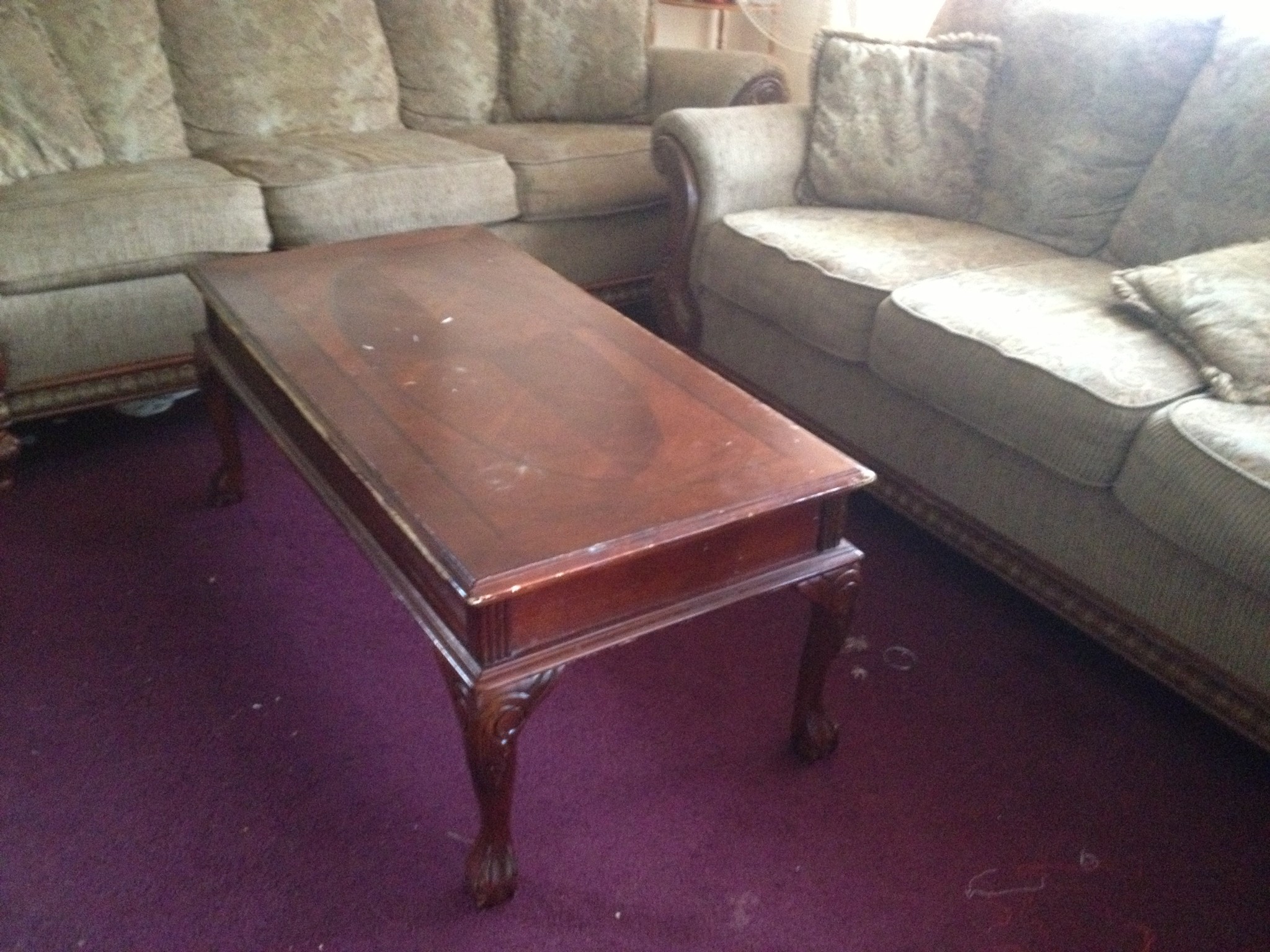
[184,225,882,907]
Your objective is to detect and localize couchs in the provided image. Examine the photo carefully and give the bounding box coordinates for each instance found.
[652,0,1270,753]
[0,0,793,416]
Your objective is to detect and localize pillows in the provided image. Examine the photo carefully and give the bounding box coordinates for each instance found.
[801,23,1002,218]
[1110,239,1270,404]
